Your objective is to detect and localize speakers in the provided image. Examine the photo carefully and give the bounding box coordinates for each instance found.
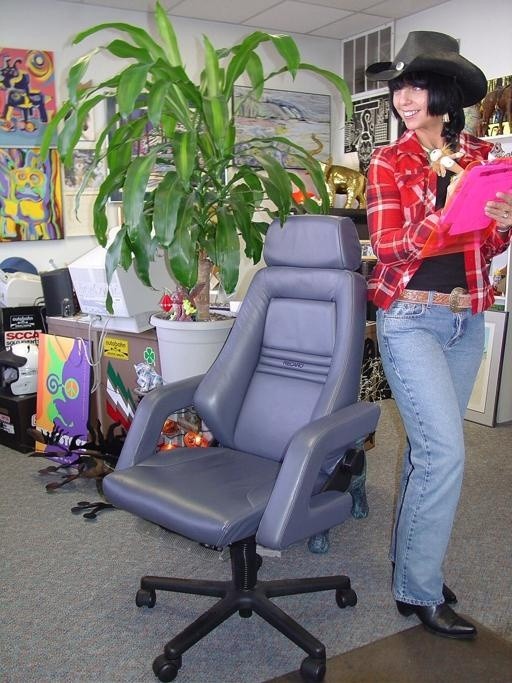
[39,268,76,316]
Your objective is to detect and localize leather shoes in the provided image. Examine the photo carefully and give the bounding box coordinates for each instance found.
[389,561,458,606]
[396,598,478,640]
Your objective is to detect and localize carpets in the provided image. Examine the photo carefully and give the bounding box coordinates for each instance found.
[255,610,512,682]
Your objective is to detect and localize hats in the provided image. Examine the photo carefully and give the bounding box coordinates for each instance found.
[362,29,487,107]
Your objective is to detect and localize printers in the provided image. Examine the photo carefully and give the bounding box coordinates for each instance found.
[0,266,44,307]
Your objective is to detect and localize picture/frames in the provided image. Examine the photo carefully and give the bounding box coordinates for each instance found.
[0,146,63,243]
[0,49,60,149]
[61,148,109,196]
[462,310,509,428]
[105,84,230,205]
[101,211,381,681]
[461,74,512,141]
[58,70,95,143]
[232,83,331,172]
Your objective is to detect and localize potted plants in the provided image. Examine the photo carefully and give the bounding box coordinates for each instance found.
[39,1,354,387]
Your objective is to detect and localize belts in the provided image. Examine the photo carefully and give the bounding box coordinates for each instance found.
[398,287,473,310]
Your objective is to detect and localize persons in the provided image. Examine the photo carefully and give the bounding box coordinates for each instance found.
[364,32,512,637]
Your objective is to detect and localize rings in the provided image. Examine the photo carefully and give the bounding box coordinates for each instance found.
[503,213,508,218]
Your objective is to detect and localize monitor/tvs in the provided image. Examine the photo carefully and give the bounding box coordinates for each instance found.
[69,225,180,334]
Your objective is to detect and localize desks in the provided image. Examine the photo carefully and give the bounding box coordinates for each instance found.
[44,316,160,456]
